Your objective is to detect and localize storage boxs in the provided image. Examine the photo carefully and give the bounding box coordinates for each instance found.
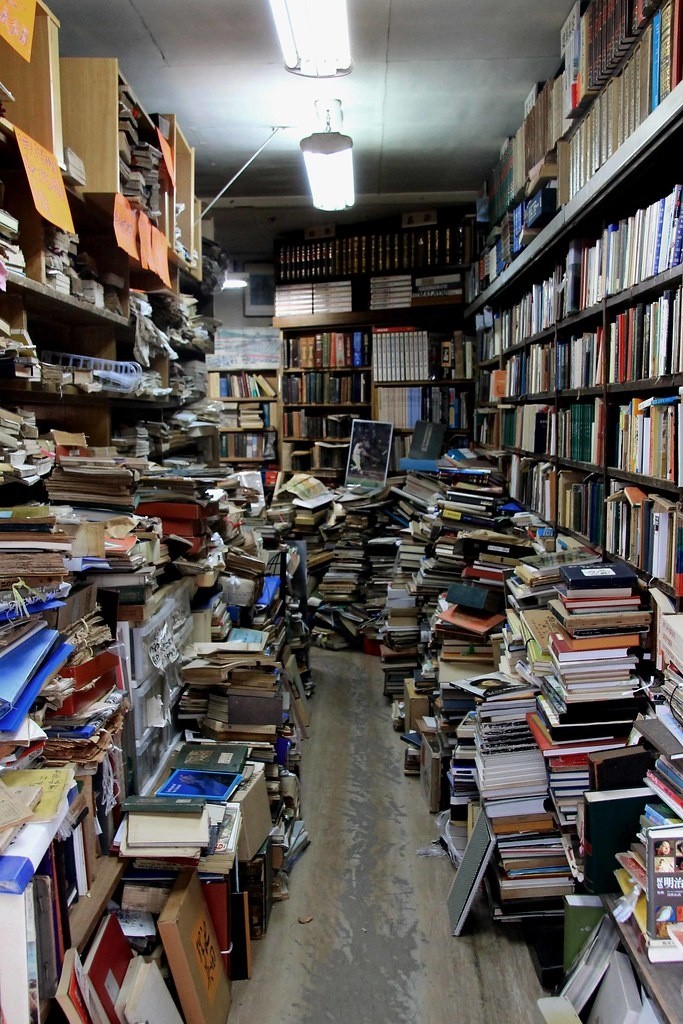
[156,867,233,1024]
[231,769,273,862]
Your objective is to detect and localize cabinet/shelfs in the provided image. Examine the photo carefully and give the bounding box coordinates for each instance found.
[0,0,683,1024]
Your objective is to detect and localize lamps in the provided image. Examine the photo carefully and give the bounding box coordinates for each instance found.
[300,97,358,210]
[269,0,355,78]
[221,271,252,291]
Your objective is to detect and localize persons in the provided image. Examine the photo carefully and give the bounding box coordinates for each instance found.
[351,442,365,476]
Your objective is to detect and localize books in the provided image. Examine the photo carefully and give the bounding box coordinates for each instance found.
[0,1,681,1024]
